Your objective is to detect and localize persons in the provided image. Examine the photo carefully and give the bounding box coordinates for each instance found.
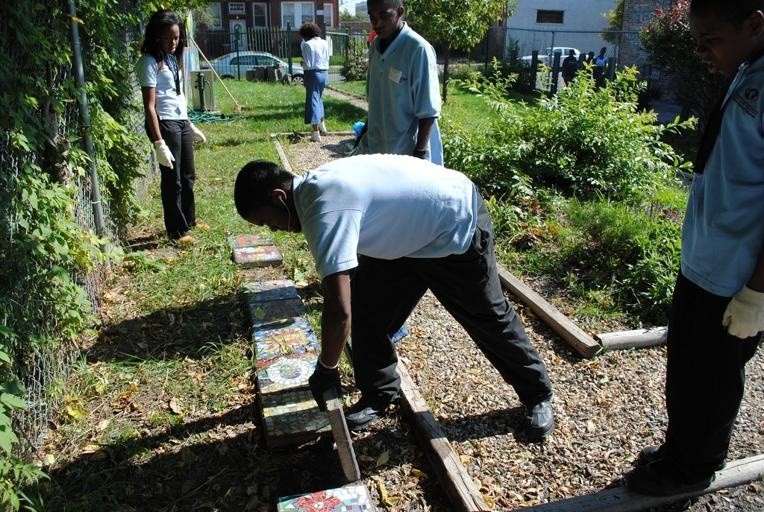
[352,0,442,347]
[299,22,332,140]
[136,9,212,247]
[561,45,611,93]
[623,1,763,499]
[234,153,557,439]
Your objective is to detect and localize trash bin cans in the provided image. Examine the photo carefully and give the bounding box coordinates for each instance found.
[246,65,285,83]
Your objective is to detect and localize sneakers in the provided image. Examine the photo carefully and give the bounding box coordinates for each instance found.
[344,399,380,430]
[627,445,726,493]
[528,401,554,436]
[310,124,327,142]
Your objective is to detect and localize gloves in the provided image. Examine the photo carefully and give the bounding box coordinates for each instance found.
[723,284,764,339]
[309,356,341,411]
[190,122,206,144]
[154,140,175,170]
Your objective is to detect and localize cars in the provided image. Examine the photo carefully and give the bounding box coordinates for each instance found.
[200,50,305,85]
[517,46,597,69]
